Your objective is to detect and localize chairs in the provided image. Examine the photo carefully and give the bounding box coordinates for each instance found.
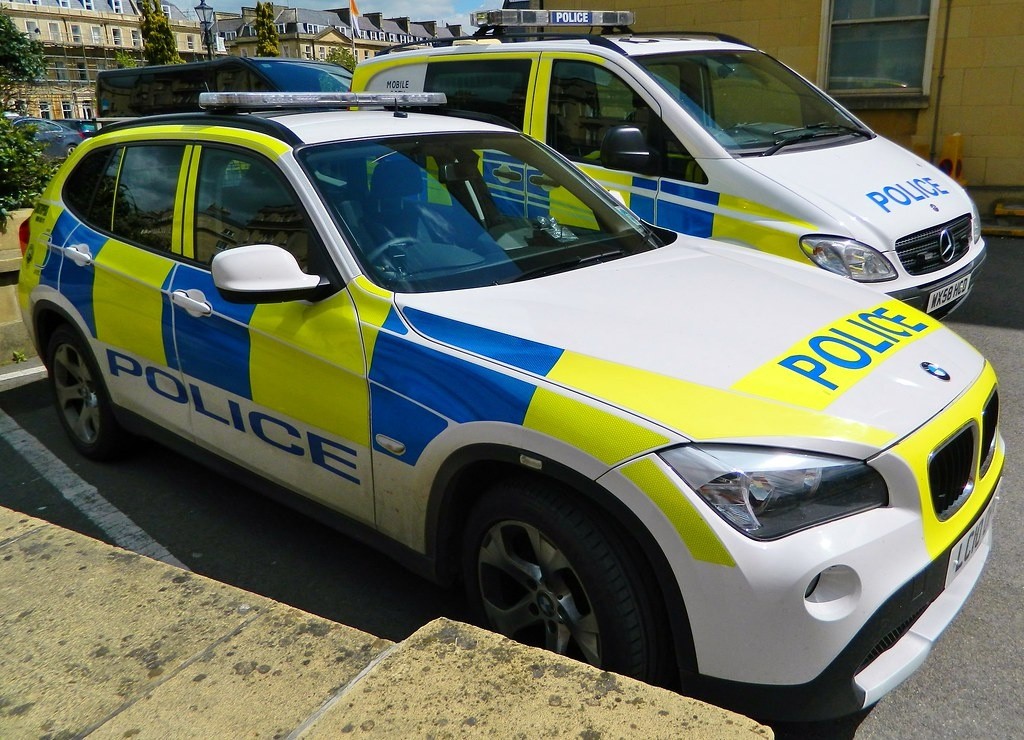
[358,157,452,247]
[556,72,609,154]
[625,91,674,143]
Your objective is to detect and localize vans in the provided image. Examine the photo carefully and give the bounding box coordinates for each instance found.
[94,54,354,123]
[350,6,987,326]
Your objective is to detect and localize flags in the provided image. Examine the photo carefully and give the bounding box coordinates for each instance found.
[350,0,359,37]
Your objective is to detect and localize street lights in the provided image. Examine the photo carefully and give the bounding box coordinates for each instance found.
[194,0,214,59]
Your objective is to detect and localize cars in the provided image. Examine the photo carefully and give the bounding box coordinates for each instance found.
[12,83,1008,722]
[1,110,100,162]
